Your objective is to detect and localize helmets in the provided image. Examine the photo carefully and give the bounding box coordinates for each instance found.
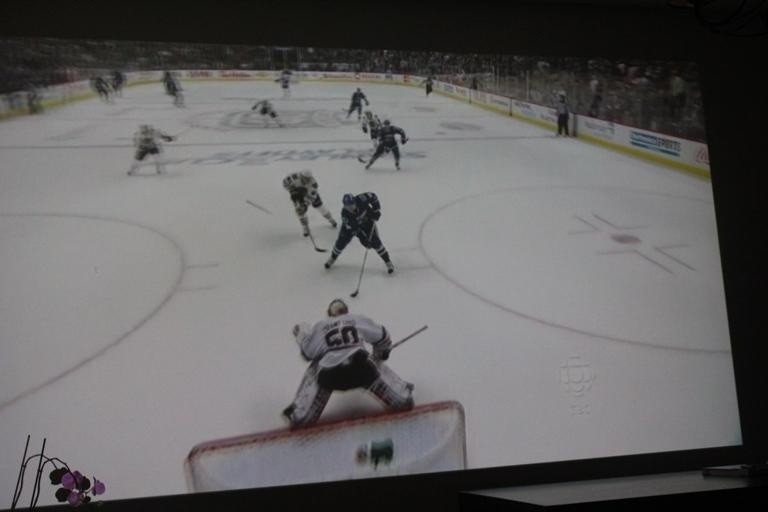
[301,169,313,178]
[329,299,348,316]
[343,194,356,206]
[383,120,390,125]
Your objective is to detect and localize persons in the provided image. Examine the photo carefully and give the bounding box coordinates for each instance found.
[325,191,393,274]
[284,297,413,427]
[2,51,705,173]
[283,167,337,237]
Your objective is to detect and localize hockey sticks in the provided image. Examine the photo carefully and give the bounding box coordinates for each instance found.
[350,220,376,298]
[300,215,327,253]
[358,137,408,163]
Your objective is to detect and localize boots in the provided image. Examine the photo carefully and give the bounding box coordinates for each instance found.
[386,259,395,273]
[303,217,337,269]
